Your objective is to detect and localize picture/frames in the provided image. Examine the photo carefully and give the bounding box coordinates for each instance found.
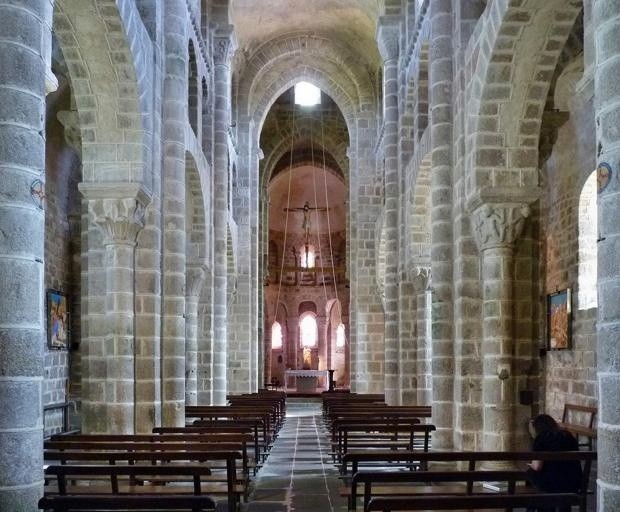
[47,288,72,351]
[546,287,573,351]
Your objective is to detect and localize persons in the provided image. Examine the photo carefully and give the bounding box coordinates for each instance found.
[524,412,588,511]
[285,202,330,239]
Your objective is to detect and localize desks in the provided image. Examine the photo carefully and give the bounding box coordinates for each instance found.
[285,369,330,394]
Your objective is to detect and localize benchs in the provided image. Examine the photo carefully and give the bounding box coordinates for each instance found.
[36,388,287,512]
[320,389,599,512]
